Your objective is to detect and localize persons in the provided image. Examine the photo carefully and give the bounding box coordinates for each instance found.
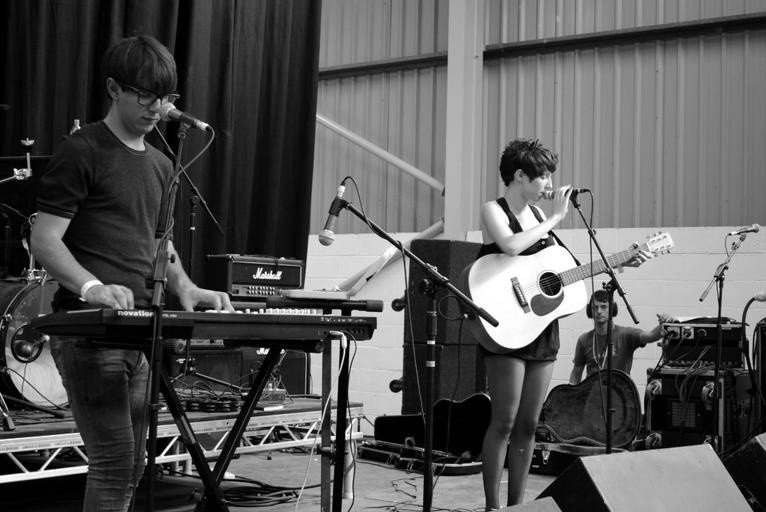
[568,290,676,389]
[473,138,656,511]
[27,36,236,512]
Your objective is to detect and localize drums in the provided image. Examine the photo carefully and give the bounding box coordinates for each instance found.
[0,275,73,411]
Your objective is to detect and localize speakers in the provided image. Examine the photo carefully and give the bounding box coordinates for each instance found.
[168,301,312,397]
[401,239,494,415]
[489,432,766,512]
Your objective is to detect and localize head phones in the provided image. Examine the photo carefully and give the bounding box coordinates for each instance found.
[586,302,617,318]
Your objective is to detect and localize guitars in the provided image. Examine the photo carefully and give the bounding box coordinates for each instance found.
[456,229,674,354]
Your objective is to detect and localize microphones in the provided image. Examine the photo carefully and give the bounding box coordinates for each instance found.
[158,102,214,133]
[15,341,35,359]
[729,223,760,236]
[545,188,588,200]
[753,290,766,301]
[318,181,346,247]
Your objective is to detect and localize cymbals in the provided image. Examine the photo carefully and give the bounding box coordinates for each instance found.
[0,154,52,159]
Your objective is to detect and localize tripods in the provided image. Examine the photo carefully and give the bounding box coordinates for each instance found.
[154,124,246,392]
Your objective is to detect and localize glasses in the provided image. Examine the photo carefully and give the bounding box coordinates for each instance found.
[124,83,180,106]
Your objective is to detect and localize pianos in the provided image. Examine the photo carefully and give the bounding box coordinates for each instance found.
[21,299,379,346]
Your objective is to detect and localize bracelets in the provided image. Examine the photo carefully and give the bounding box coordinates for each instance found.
[79,276,105,304]
